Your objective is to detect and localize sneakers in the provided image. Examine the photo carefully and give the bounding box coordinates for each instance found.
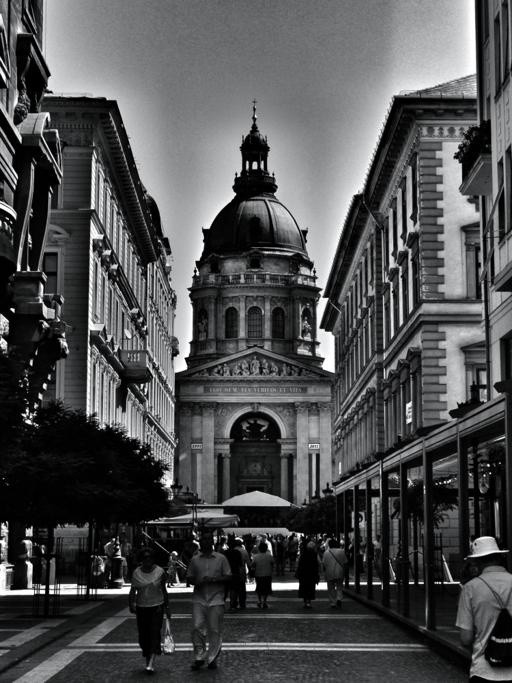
[144,665,155,672]
[190,660,217,671]
[232,602,268,611]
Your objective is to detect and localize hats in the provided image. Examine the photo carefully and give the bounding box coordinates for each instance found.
[463,535,510,563]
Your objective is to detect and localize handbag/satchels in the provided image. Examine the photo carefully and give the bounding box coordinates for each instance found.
[162,618,175,657]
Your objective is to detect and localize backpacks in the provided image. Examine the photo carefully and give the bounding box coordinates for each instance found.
[475,575,512,668]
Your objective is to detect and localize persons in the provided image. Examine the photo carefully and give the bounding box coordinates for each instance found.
[185,533,232,670]
[456,536,512,683]
[91,529,382,610]
[201,355,309,375]
[129,551,171,673]
